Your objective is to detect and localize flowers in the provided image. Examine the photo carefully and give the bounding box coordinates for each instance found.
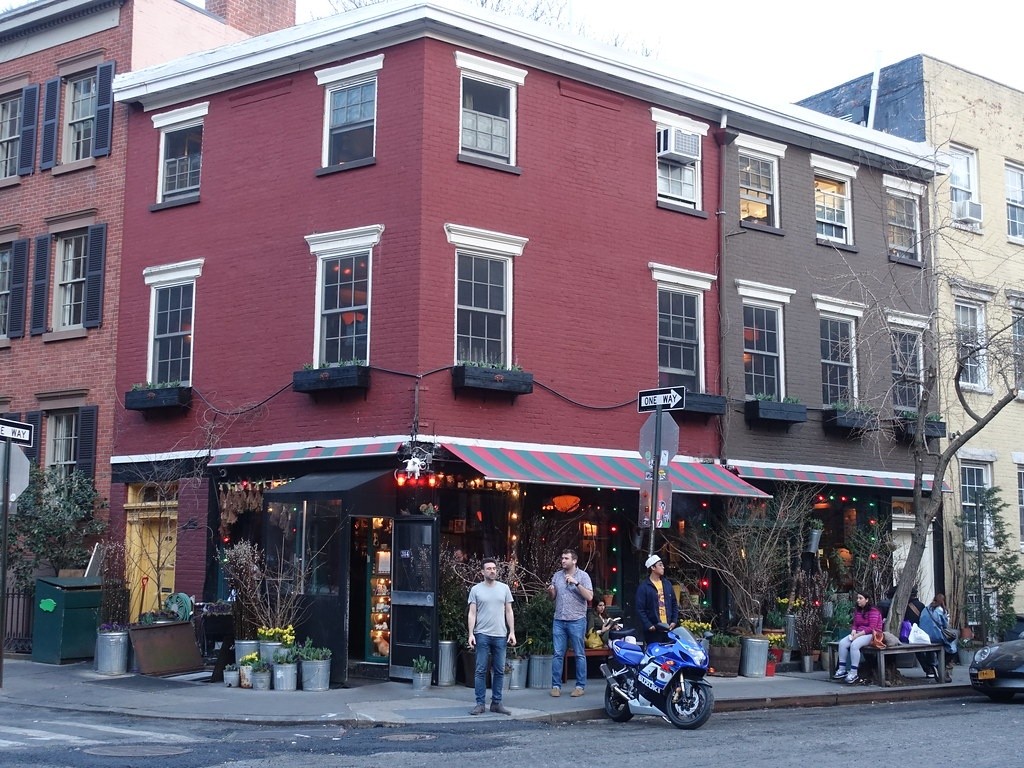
[680,619,712,638]
[203,504,355,664]
[765,569,832,655]
[96,537,168,631]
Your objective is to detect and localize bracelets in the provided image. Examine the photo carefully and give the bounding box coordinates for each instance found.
[574,581,580,586]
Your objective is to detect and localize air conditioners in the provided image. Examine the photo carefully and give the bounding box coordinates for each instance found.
[954,199,982,223]
[658,126,702,164]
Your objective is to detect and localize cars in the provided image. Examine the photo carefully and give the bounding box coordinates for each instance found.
[968,629,1024,703]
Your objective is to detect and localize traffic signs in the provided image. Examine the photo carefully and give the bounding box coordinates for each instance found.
[0,419,34,448]
[637,385,685,413]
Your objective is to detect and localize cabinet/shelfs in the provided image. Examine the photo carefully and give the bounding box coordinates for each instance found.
[364,572,391,663]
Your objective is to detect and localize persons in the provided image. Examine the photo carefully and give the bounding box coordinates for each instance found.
[919,594,957,682]
[467,559,516,715]
[832,592,882,683]
[587,597,622,643]
[546,549,593,697]
[880,587,936,677]
[636,555,678,646]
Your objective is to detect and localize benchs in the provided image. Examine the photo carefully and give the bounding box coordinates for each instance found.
[563,647,612,683]
[827,641,946,687]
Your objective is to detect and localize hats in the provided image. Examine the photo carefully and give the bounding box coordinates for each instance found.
[644,554,661,569]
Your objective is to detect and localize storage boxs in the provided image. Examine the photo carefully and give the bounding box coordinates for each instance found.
[375,551,389,573]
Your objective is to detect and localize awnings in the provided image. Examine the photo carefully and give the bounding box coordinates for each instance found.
[205,442,402,467]
[732,465,954,493]
[441,442,774,500]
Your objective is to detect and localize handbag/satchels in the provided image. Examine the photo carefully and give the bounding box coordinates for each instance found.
[900,620,912,643]
[870,630,885,649]
[943,628,958,643]
[908,623,931,645]
[584,627,603,650]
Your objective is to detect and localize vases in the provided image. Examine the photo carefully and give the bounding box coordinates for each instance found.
[696,638,709,651]
[684,392,947,439]
[774,624,782,629]
[801,655,813,674]
[587,599,593,606]
[97,613,282,681]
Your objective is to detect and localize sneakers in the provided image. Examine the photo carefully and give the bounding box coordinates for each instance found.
[834,669,847,678]
[845,673,858,683]
[926,671,935,678]
[490,702,512,714]
[551,688,561,697]
[944,674,953,682]
[470,705,486,715]
[569,687,585,697]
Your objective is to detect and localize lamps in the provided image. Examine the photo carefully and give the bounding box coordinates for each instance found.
[551,496,581,513]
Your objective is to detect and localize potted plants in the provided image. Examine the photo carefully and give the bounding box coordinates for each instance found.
[293,356,370,392]
[708,631,792,676]
[414,564,554,690]
[596,587,616,605]
[124,383,193,408]
[222,636,332,691]
[804,518,856,672]
[452,362,535,395]
[956,638,976,667]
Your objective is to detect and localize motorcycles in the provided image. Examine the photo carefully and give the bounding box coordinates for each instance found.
[598,622,714,730]
